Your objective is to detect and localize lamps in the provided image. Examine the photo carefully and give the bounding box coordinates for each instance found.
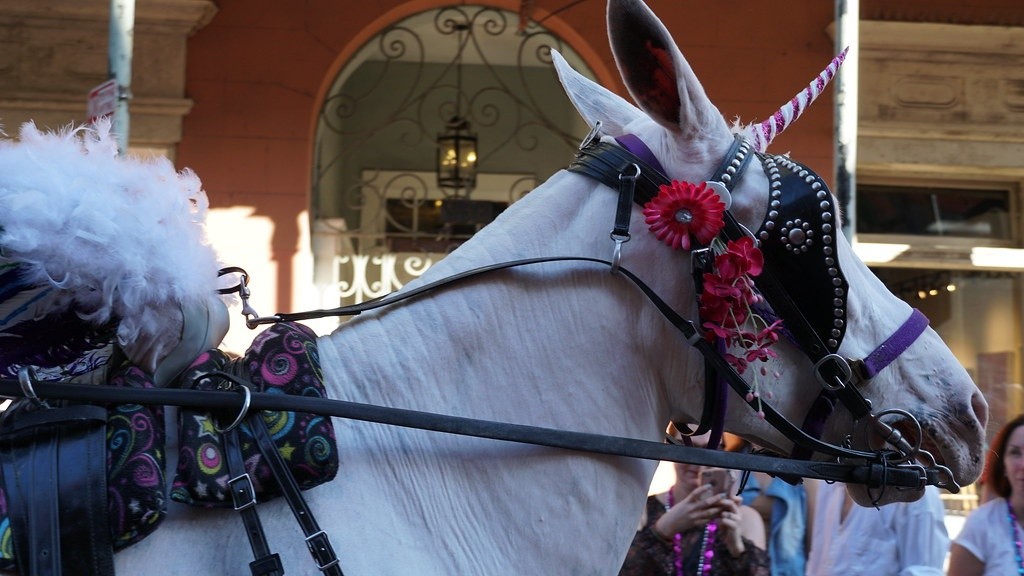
[434,22,480,204]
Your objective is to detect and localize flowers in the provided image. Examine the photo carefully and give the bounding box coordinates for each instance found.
[699,236,784,419]
[643,181,726,249]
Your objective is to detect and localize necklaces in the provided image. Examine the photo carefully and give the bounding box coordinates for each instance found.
[1006,499,1024,576]
[665,486,718,576]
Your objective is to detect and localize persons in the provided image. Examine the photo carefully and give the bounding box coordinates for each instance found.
[945,415,1024,576]
[805,479,949,576]
[618,423,769,576]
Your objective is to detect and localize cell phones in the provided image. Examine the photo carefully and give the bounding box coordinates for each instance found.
[699,467,743,502]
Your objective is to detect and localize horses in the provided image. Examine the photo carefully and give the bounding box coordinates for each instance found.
[0,0,985,576]
